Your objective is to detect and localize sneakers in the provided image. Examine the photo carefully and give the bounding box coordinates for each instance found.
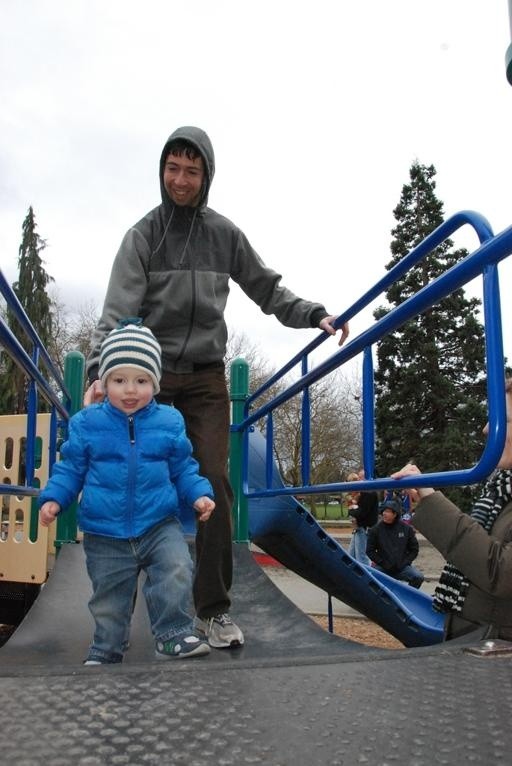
[155,632,212,659]
[194,612,245,648]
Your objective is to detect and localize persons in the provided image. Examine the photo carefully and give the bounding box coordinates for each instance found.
[366,499,425,590]
[79,123,351,652]
[345,470,380,567]
[345,473,363,533]
[382,488,418,525]
[388,376,511,645]
[36,316,218,668]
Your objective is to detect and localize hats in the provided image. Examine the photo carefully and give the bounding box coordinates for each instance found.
[98,318,162,396]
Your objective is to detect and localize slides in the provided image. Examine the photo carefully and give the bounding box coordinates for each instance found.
[249,423,449,647]
